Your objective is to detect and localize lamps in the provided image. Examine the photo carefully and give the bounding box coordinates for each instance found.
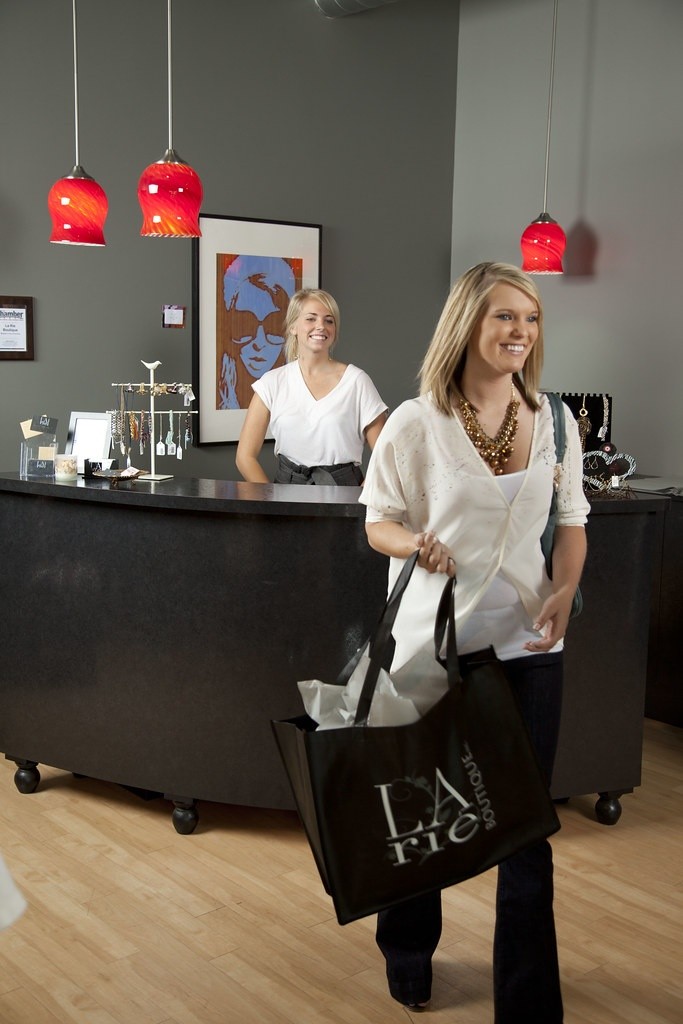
[520,0,567,275]
[47,0,109,248]
[136,0,203,240]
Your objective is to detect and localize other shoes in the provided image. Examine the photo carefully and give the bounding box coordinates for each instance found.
[386,957,433,1008]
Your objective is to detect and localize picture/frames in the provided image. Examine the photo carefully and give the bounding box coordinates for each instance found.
[192,214,324,449]
[64,410,113,475]
[0,296,34,360]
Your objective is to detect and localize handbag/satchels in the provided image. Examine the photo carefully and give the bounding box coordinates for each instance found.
[270,548,562,926]
[538,391,584,620]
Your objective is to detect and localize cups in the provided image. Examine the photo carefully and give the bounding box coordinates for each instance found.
[55,454,77,482]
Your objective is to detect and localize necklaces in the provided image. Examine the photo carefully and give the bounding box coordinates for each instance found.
[448,380,522,475]
[121,387,133,466]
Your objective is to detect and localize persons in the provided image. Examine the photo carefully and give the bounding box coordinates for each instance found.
[359,263,593,1024]
[235,289,390,488]
